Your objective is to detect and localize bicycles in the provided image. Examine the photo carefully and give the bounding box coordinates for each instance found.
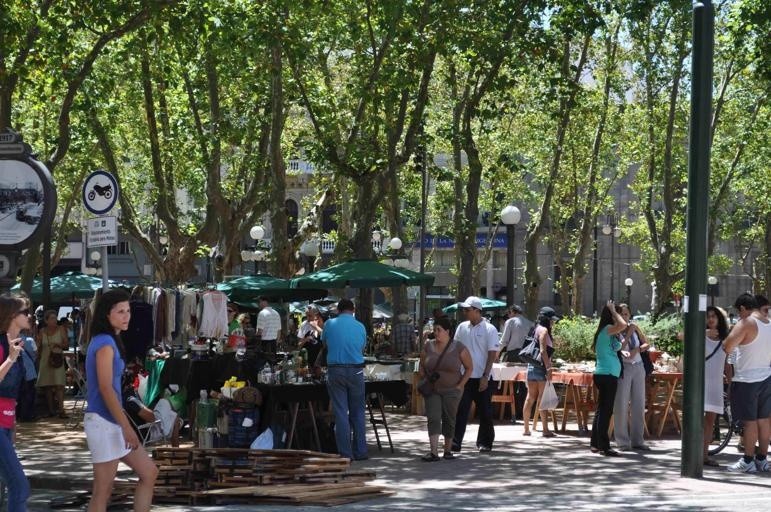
[703,378,741,457]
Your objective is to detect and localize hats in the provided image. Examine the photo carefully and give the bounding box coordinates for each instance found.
[540,306,559,320]
[458,296,483,310]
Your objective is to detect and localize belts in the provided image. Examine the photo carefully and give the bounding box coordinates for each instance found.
[329,363,365,368]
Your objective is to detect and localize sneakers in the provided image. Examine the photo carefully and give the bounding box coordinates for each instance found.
[59,414,69,418]
[524,432,530,436]
[47,413,57,417]
[704,459,718,466]
[479,445,492,452]
[590,443,652,456]
[754,459,769,472]
[442,443,461,451]
[726,458,756,473]
[543,432,556,437]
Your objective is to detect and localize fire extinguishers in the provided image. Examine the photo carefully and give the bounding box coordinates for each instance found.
[674,287,684,306]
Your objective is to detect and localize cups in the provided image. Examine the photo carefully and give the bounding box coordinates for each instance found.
[200,390,222,403]
[379,355,392,361]
[653,356,678,374]
[552,359,595,373]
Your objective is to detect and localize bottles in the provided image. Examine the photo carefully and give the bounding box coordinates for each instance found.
[258,348,328,385]
[209,337,218,356]
[727,345,737,365]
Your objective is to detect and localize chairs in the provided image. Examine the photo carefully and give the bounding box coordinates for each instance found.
[66,367,87,429]
[123,409,167,450]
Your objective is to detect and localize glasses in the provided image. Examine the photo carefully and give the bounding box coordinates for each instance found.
[18,309,29,316]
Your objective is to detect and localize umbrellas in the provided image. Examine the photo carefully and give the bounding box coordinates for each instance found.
[207,270,328,304]
[289,259,435,287]
[10,270,137,396]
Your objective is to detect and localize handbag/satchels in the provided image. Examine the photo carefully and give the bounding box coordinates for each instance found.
[418,372,440,397]
[48,351,62,367]
[640,351,654,375]
[234,387,262,406]
[517,339,555,370]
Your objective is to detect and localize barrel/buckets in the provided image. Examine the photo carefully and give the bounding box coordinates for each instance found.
[227,404,260,447]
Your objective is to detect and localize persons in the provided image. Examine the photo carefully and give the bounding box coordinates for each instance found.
[677,293,771,466]
[589,300,650,455]
[1,287,559,512]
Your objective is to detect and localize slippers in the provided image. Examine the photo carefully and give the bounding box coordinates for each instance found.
[422,452,440,462]
[444,452,456,459]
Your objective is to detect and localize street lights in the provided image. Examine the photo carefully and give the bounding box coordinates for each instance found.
[361,218,402,269]
[624,277,634,325]
[239,226,269,280]
[502,204,521,315]
[299,240,318,307]
[602,206,623,314]
[84,252,103,283]
[707,275,719,307]
